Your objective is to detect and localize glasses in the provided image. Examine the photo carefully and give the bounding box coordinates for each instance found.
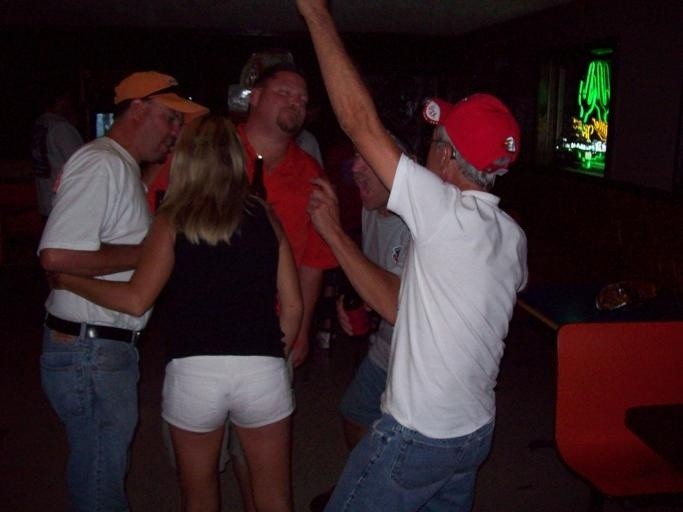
[423,135,455,158]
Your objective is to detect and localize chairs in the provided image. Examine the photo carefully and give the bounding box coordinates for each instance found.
[555,321,682,494]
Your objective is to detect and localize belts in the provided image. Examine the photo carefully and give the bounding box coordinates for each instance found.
[40,310,140,343]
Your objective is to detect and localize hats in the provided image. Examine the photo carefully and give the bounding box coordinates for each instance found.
[421,91,520,170]
[110,69,209,118]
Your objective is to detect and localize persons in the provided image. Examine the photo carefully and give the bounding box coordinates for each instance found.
[49,112,305,511]
[305,111,417,511]
[36,69,209,512]
[33,77,86,229]
[147,60,342,512]
[299,1,529,512]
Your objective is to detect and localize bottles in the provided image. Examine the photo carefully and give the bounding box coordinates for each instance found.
[343,285,373,348]
[250,156,267,201]
